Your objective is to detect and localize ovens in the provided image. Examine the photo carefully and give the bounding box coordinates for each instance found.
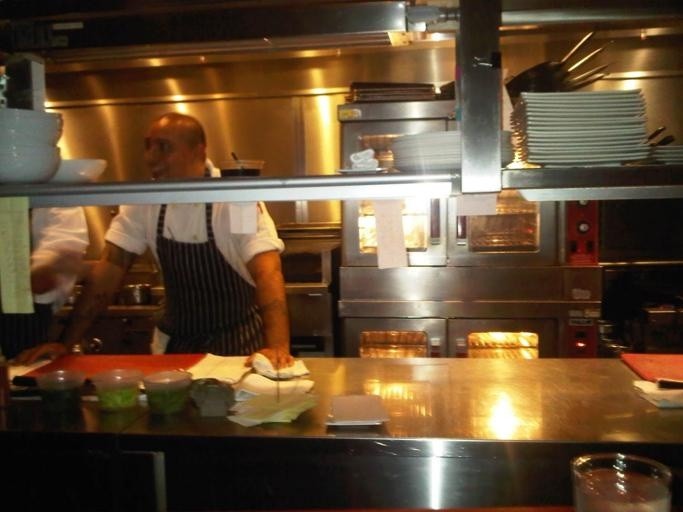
[337,99,602,358]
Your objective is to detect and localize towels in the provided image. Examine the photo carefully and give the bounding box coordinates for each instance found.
[243,374,315,394]
[254,354,310,379]
[632,379,683,408]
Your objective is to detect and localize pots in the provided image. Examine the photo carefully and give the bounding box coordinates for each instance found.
[501,29,615,104]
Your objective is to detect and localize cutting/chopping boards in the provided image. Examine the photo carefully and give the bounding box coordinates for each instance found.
[14,353,207,385]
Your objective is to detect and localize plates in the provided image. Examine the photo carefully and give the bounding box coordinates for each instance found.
[325,411,382,427]
[652,143,683,164]
[337,168,387,175]
[389,131,510,172]
[575,453,671,512]
[509,88,652,168]
[332,395,390,423]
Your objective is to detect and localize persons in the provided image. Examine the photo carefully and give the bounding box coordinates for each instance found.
[9,206,104,364]
[14,112,295,366]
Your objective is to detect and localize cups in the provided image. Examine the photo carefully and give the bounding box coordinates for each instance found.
[91,369,140,411]
[37,371,85,413]
[143,371,192,415]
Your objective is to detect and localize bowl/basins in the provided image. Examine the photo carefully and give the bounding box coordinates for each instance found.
[217,158,266,177]
[51,160,108,182]
[0,106,64,184]
